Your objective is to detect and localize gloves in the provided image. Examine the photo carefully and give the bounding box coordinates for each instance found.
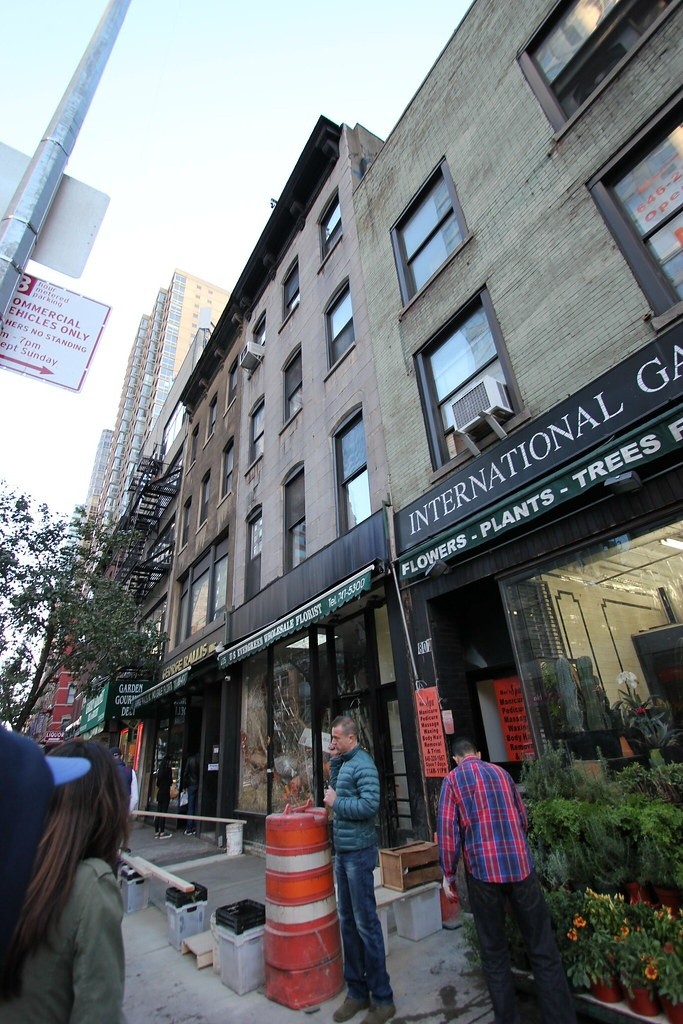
[443,875,458,904]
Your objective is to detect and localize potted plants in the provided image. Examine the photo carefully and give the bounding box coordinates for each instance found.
[621,712,682,765]
[538,656,625,760]
[583,793,683,919]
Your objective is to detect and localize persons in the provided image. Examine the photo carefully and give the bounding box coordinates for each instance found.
[322,715,397,1024]
[0,738,132,1024]
[152,753,172,840]
[436,739,579,1024]
[0,725,55,956]
[109,746,140,853]
[181,745,200,835]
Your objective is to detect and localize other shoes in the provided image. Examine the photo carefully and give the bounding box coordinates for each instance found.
[193,831,196,834]
[119,847,131,854]
[184,830,192,835]
[155,832,159,839]
[160,832,172,839]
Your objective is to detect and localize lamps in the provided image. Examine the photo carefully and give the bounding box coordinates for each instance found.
[425,559,448,578]
[603,471,642,496]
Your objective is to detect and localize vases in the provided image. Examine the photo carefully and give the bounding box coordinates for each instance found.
[586,976,683,1024]
[621,726,645,755]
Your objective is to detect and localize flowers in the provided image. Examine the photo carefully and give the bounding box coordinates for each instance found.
[617,670,683,726]
[568,886,683,1006]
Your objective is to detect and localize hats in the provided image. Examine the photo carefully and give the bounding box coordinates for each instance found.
[110,747,121,756]
[44,755,92,787]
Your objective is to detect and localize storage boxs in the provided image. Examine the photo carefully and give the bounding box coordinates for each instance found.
[165,881,208,951]
[378,838,442,893]
[121,865,150,913]
[210,898,266,995]
[392,885,442,940]
[377,905,392,957]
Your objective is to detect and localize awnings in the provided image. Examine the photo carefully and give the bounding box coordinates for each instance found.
[127,650,219,710]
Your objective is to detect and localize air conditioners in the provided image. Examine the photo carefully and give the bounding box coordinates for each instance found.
[449,376,514,442]
[240,342,264,369]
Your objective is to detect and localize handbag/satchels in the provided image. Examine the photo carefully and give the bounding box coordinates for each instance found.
[180,788,188,807]
[170,783,180,800]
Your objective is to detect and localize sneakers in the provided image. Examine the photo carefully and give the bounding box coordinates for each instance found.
[333,997,370,1022]
[361,1003,397,1024]
